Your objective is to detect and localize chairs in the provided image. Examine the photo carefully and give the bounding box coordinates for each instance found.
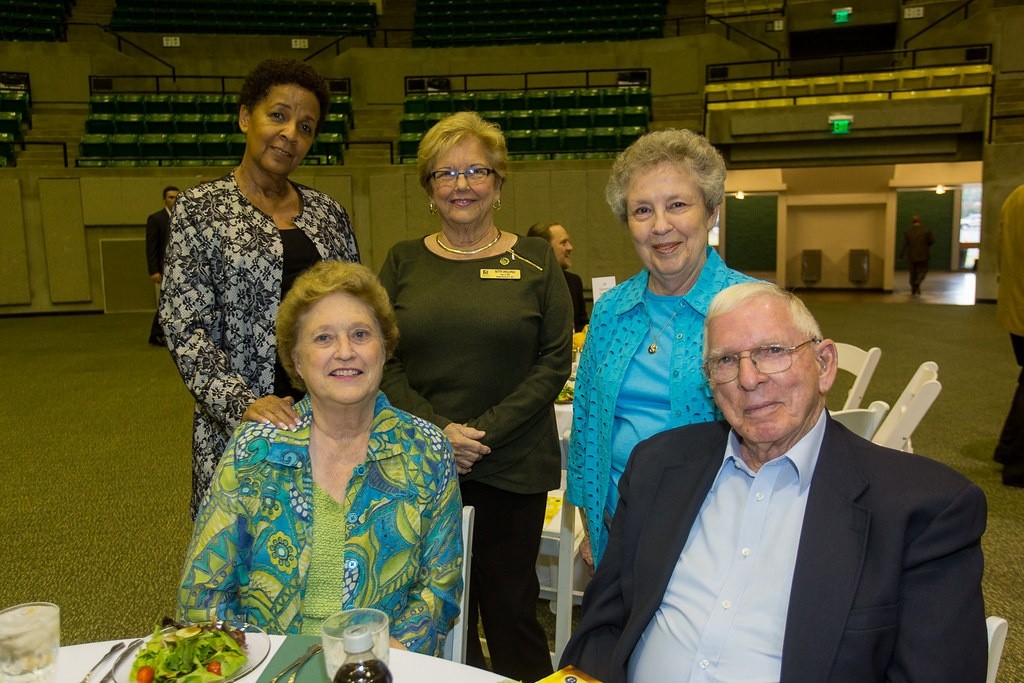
[444,432,585,673]
[829,342,941,454]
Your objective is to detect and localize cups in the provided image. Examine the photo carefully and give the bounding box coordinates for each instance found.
[0,602,63,683]
[320,608,390,680]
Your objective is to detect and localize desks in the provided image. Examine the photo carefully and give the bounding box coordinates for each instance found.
[60,635,516,683]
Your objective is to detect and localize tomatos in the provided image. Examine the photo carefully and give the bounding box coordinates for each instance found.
[208,662,221,674]
[137,666,153,683]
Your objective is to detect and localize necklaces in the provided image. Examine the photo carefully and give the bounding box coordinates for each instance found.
[645,288,676,353]
[436,228,502,255]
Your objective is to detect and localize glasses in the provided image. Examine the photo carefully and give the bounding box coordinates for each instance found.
[428,167,498,186]
[701,338,820,384]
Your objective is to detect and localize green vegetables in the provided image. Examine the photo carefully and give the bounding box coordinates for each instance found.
[129,623,248,683]
[558,385,575,401]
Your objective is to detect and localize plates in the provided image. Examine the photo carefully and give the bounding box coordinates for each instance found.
[555,399,573,405]
[112,619,271,683]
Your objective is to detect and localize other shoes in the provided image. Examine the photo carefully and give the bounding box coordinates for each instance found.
[149,335,167,346]
[1004,464,1024,486]
[994,449,1005,463]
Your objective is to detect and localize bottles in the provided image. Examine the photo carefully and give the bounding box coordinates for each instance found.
[332,624,394,683]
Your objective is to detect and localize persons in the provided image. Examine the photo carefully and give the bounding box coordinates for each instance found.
[555,282,988,683]
[903,214,935,295]
[993,184,1024,488]
[566,129,777,576]
[156,58,360,524]
[176,260,464,659]
[379,112,574,683]
[145,186,180,349]
[527,221,590,333]
